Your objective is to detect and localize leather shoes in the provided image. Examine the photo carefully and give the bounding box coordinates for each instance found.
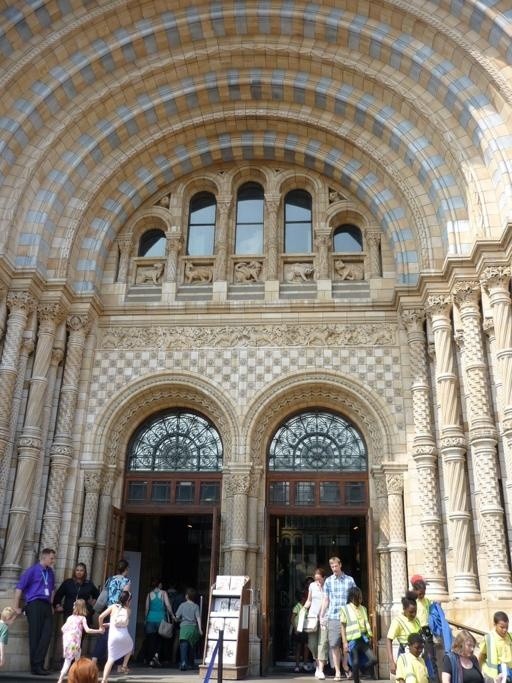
[32,667,49,675]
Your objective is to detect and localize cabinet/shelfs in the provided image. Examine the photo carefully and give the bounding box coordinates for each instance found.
[199,574,254,679]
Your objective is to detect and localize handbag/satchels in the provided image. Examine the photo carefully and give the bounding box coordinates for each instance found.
[158,619,173,639]
[304,614,318,632]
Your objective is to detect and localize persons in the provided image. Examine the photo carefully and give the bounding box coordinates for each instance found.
[13,549,56,676]
[287,556,511,682]
[0,607,16,668]
[53,562,100,666]
[143,579,175,667]
[56,598,106,683]
[91,559,131,673]
[98,591,133,683]
[174,590,203,670]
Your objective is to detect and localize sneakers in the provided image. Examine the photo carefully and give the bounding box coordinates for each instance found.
[315,667,352,681]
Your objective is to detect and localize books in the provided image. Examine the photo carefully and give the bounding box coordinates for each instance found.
[204,574,244,667]
